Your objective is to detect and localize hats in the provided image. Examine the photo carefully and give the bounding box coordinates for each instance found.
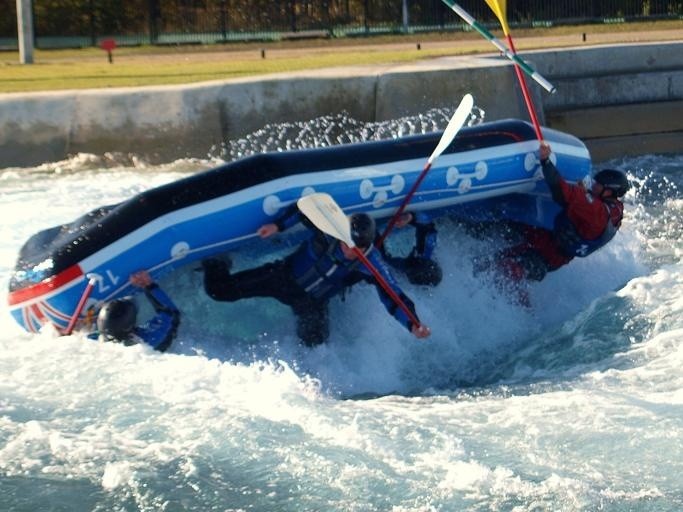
[351,213,375,247]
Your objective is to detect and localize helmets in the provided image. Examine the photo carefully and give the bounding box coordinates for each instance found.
[407,256,443,286]
[594,169,629,197]
[96,296,137,334]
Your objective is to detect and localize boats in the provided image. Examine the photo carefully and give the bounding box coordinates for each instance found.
[7,117,594,338]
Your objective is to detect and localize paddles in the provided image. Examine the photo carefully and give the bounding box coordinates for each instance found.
[486,0,543,141]
[375,93,474,248]
[297,192,419,328]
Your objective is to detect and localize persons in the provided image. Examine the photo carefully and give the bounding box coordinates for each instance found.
[86,269,182,354]
[193,201,432,347]
[373,209,444,287]
[448,141,632,319]
